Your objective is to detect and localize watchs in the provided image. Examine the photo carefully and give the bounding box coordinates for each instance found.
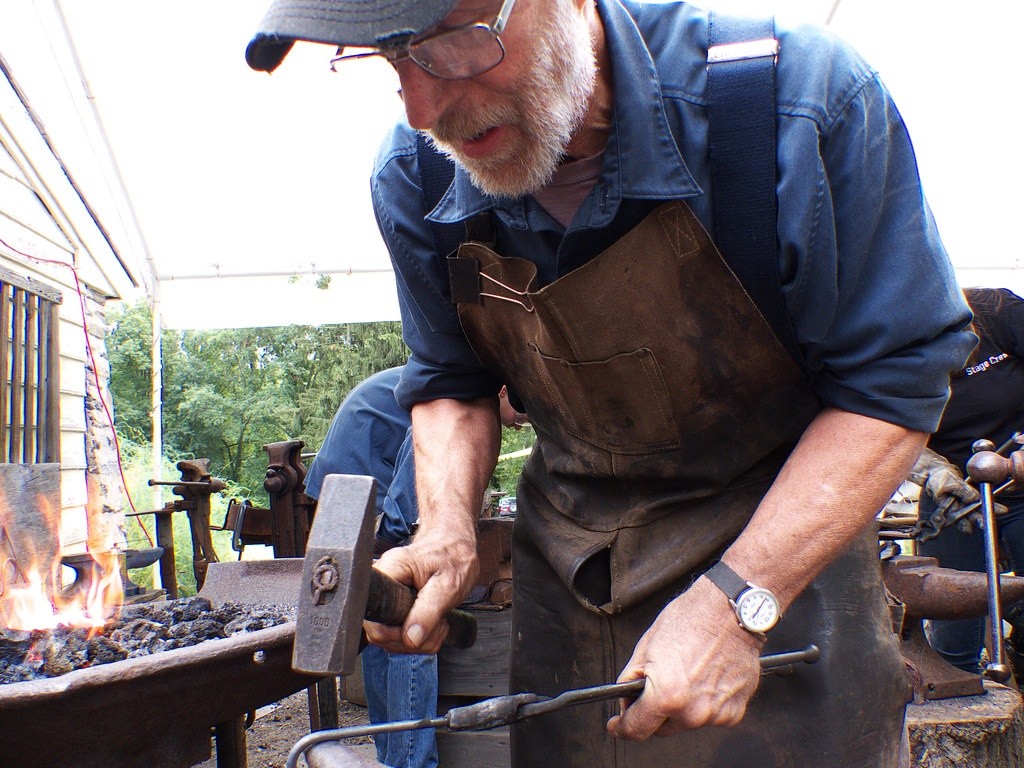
[702,560,782,640]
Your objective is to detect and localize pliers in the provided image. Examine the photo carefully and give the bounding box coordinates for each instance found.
[910,432,1020,544]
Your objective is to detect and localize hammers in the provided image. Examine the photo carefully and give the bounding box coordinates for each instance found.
[293,473,477,677]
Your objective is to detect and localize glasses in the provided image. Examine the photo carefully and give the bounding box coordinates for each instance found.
[330,0,515,95]
[515,412,533,426]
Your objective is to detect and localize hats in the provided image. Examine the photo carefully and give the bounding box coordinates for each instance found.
[245,1,460,73]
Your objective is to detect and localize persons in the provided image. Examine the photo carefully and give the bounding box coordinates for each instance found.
[303,364,534,768]
[906,287,1024,701]
[244,0,981,768]
